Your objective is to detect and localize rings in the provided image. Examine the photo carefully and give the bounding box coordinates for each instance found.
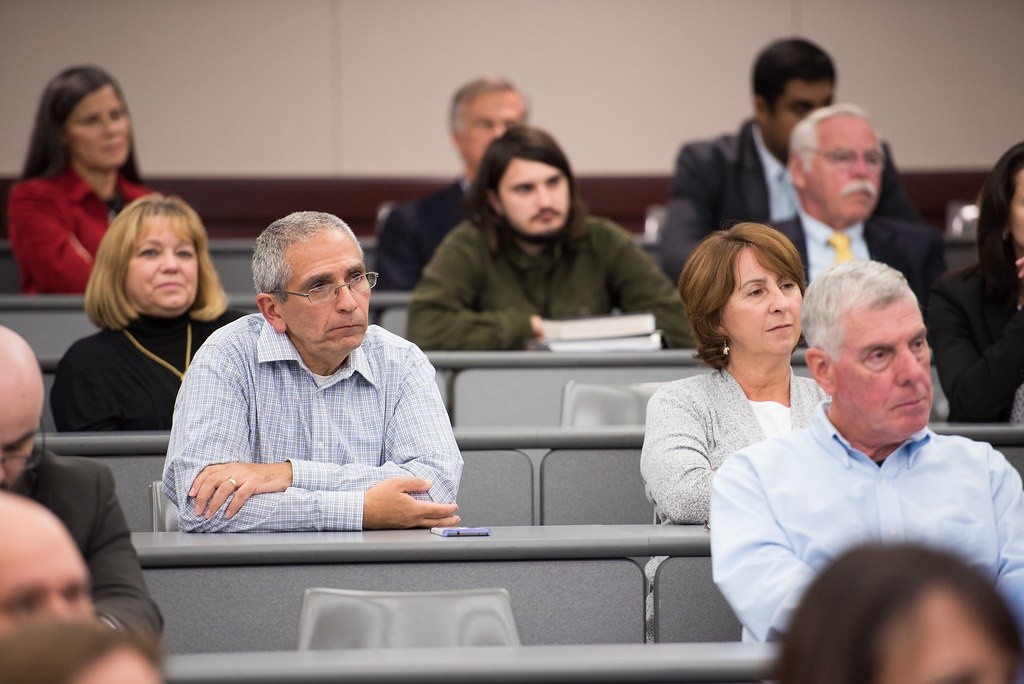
[228,479,237,488]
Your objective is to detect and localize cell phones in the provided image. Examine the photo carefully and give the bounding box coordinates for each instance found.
[430,526,491,537]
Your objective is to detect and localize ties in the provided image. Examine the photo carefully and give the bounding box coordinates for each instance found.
[827,232,854,265]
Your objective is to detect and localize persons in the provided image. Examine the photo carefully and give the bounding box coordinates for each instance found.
[772,538,1024,684]
[0,623,170,684]
[161,210,465,534]
[7,63,165,294]
[370,78,529,294]
[405,124,701,351]
[710,259,1024,645]
[639,221,833,644]
[658,37,953,288]
[0,325,166,650]
[0,491,97,639]
[48,191,239,433]
[917,140,1024,424]
[768,101,949,347]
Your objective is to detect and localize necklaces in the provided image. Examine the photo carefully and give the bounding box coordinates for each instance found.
[122,322,192,383]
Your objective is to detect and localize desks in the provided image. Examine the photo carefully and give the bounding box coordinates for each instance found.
[0,241,1024,684]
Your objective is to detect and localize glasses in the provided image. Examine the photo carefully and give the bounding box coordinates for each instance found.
[808,147,885,176]
[0,414,45,473]
[270,268,380,305]
